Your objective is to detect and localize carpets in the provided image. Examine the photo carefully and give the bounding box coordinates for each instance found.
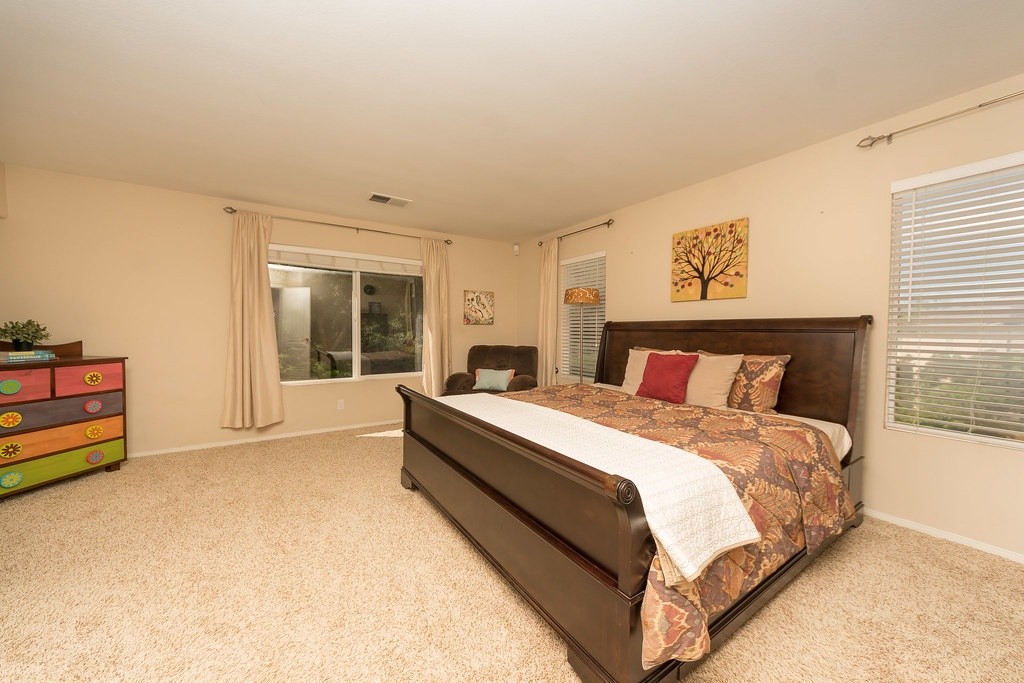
[0,422,1024,683]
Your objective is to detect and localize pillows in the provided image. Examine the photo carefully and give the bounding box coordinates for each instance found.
[620,345,791,416]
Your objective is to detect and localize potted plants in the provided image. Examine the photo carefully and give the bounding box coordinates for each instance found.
[0,320,52,352]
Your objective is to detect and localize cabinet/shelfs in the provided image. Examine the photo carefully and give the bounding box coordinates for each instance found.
[0,340,127,501]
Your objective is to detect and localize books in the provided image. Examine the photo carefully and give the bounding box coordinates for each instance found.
[0,351,55,362]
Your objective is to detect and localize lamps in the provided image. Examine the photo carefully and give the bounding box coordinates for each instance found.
[563,287,600,385]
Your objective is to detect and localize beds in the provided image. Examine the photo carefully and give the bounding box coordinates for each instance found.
[317,349,416,378]
[395,315,873,683]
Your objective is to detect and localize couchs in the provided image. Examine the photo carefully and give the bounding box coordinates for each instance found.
[441,344,538,397]
